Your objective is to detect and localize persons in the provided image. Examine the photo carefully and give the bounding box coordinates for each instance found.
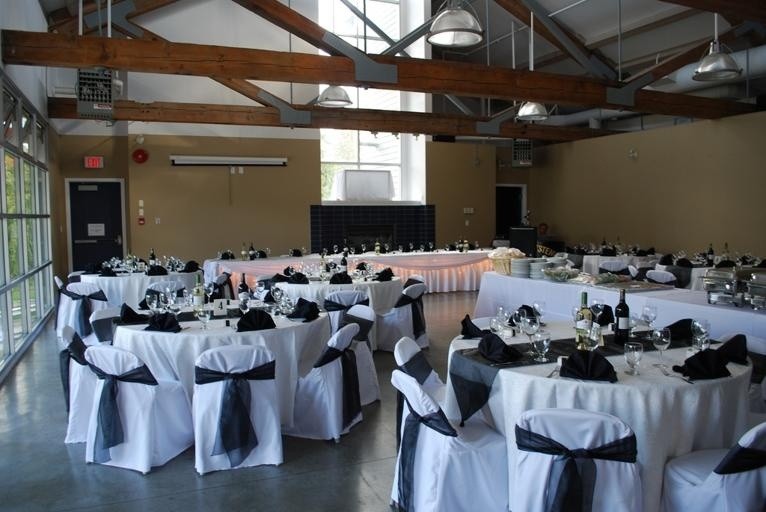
[539,222,548,235]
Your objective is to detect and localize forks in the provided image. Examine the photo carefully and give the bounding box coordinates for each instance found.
[658,366,697,385]
[547,365,564,379]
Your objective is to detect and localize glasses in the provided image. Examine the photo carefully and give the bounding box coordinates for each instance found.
[168,155,289,167]
[425,0,485,48]
[313,85,354,108]
[513,11,551,121]
[692,12,743,81]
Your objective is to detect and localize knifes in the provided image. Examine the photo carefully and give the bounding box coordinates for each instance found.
[77,67,114,120]
[512,138,533,168]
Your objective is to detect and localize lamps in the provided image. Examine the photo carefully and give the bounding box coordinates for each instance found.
[663,421,766,512]
[386,368,507,511]
[324,290,369,336]
[513,408,644,512]
[66,282,111,334]
[291,323,361,445]
[393,336,444,389]
[54,276,69,345]
[403,274,424,288]
[340,304,380,404]
[58,324,98,444]
[720,332,766,413]
[377,283,429,354]
[84,344,194,476]
[192,344,285,476]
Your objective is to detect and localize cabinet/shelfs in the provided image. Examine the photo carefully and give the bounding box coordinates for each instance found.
[150,248,156,267]
[193,274,204,318]
[238,273,249,304]
[249,242,256,261]
[576,292,591,346]
[340,254,347,274]
[615,288,630,344]
[459,235,463,252]
[319,253,326,277]
[723,241,729,260]
[708,243,714,266]
[376,238,380,254]
[241,242,248,261]
[126,249,133,267]
[616,236,621,253]
[602,238,607,249]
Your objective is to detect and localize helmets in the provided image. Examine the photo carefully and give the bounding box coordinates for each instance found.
[509,259,533,278]
[593,283,675,294]
[547,256,565,267]
[529,262,552,280]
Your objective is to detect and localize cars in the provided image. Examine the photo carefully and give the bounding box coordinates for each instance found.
[534,330,551,362]
[512,309,526,326]
[533,300,548,319]
[652,328,671,365]
[420,244,424,253]
[409,242,413,254]
[145,294,158,311]
[398,245,403,254]
[384,243,389,254]
[159,291,170,307]
[429,241,433,252]
[196,305,210,332]
[361,244,366,255]
[203,281,214,304]
[255,282,265,304]
[522,317,539,355]
[489,318,502,333]
[333,244,338,255]
[589,299,605,323]
[624,342,643,377]
[691,318,710,352]
[642,306,657,325]
[350,247,355,255]
